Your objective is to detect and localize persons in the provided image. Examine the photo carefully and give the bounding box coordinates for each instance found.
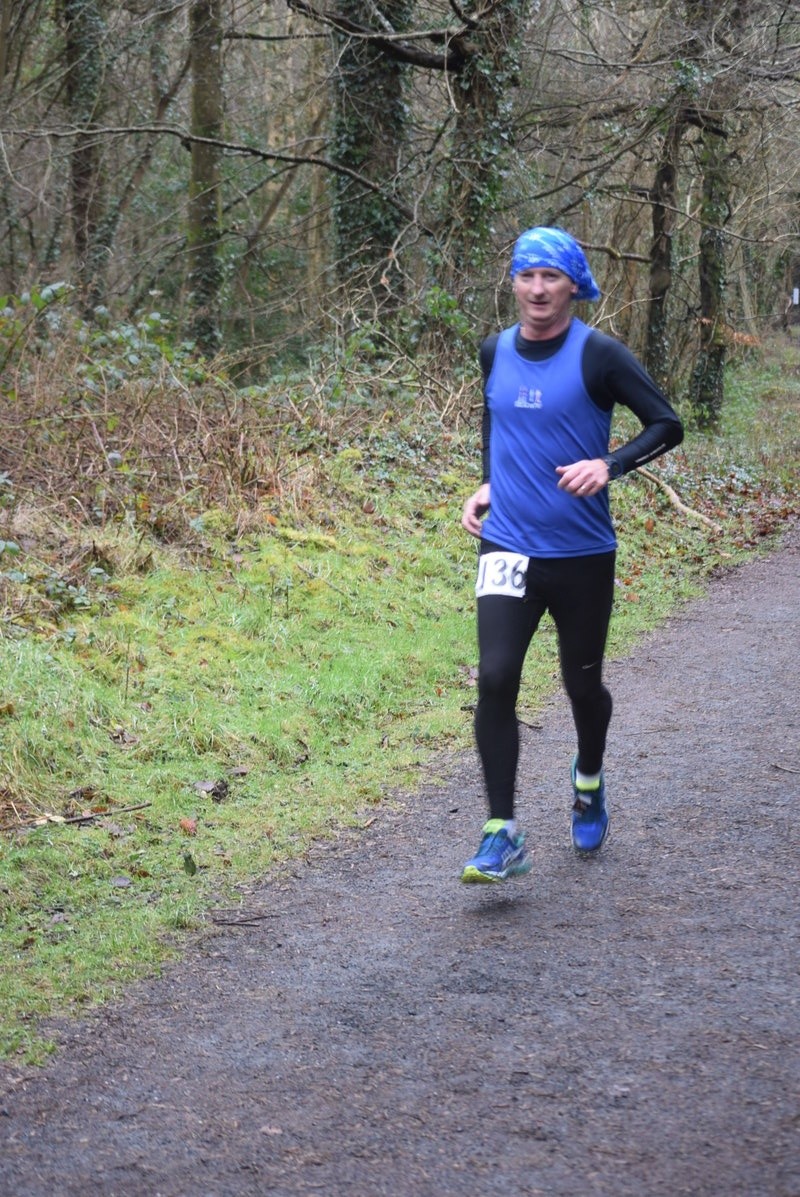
[458,222,686,882]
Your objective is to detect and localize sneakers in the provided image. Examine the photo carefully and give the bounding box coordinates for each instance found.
[570,751,610,853]
[460,818,533,884]
